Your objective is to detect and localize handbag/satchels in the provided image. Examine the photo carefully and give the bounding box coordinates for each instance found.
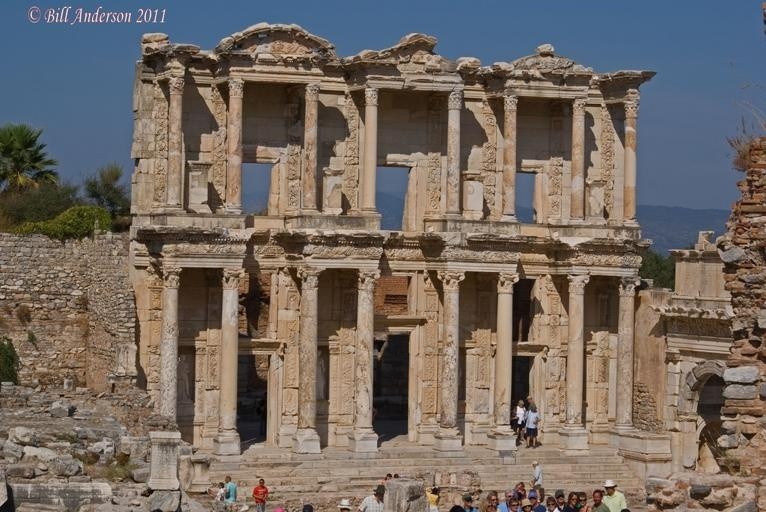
[512,407,518,420]
[225,485,231,499]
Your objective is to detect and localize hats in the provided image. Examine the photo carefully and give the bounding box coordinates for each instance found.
[555,491,564,498]
[517,400,525,406]
[521,499,533,509]
[528,491,538,500]
[601,478,617,488]
[530,461,538,467]
[336,498,353,510]
[528,403,537,410]
[525,396,532,400]
[372,484,385,494]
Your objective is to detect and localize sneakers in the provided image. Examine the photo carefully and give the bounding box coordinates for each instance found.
[518,436,536,448]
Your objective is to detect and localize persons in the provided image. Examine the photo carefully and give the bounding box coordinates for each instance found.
[250,477,270,511]
[337,473,632,511]
[528,459,544,493]
[273,504,288,512]
[221,474,237,505]
[524,402,541,448]
[215,481,225,505]
[526,396,535,421]
[511,399,527,440]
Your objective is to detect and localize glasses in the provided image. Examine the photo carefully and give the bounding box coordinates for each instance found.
[511,503,520,506]
[571,498,578,501]
[579,498,587,502]
[547,503,555,506]
[490,498,499,502]
[487,504,493,507]
[507,495,514,497]
